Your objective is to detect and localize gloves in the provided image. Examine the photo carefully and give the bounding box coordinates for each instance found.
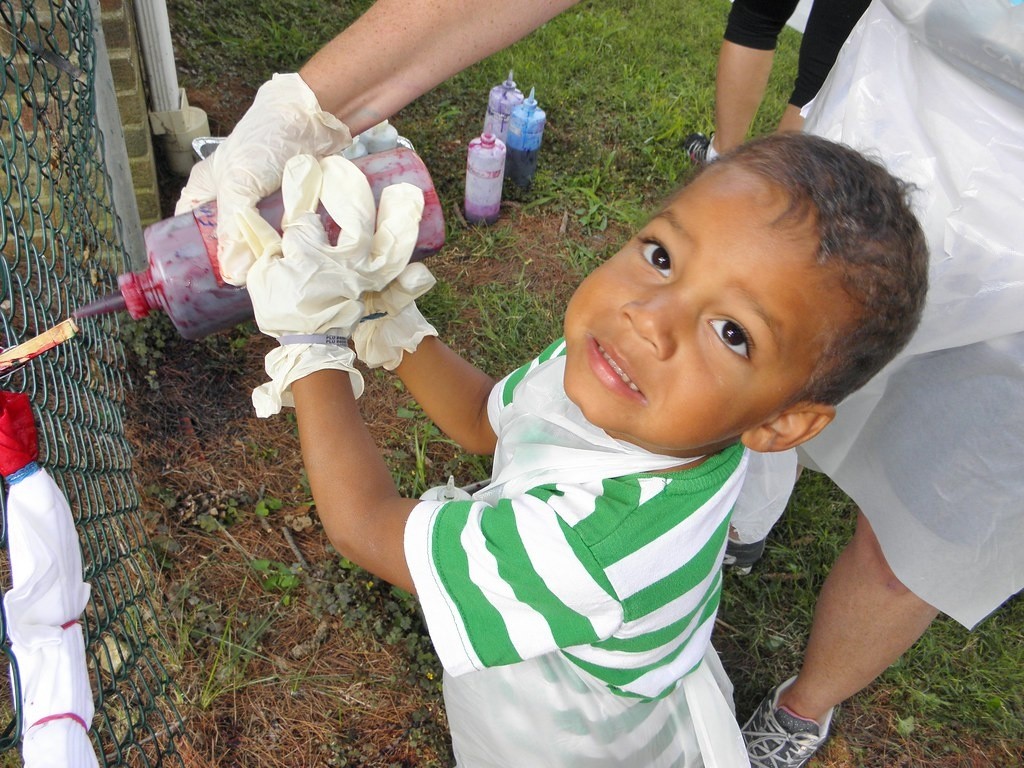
[351,262,437,372]
[234,155,425,419]
[174,71,352,288]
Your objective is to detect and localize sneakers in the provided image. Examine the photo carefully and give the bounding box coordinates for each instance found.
[740,674,836,768]
[684,133,710,164]
[722,537,764,576]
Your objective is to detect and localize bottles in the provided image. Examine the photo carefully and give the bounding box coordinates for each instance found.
[464,121,506,225]
[483,69,525,145]
[505,86,546,186]
[71,146,447,338]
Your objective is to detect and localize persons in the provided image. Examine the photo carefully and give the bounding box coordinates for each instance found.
[244,130,929,768]
[676,0,874,171]
[170,2,1024,767]
